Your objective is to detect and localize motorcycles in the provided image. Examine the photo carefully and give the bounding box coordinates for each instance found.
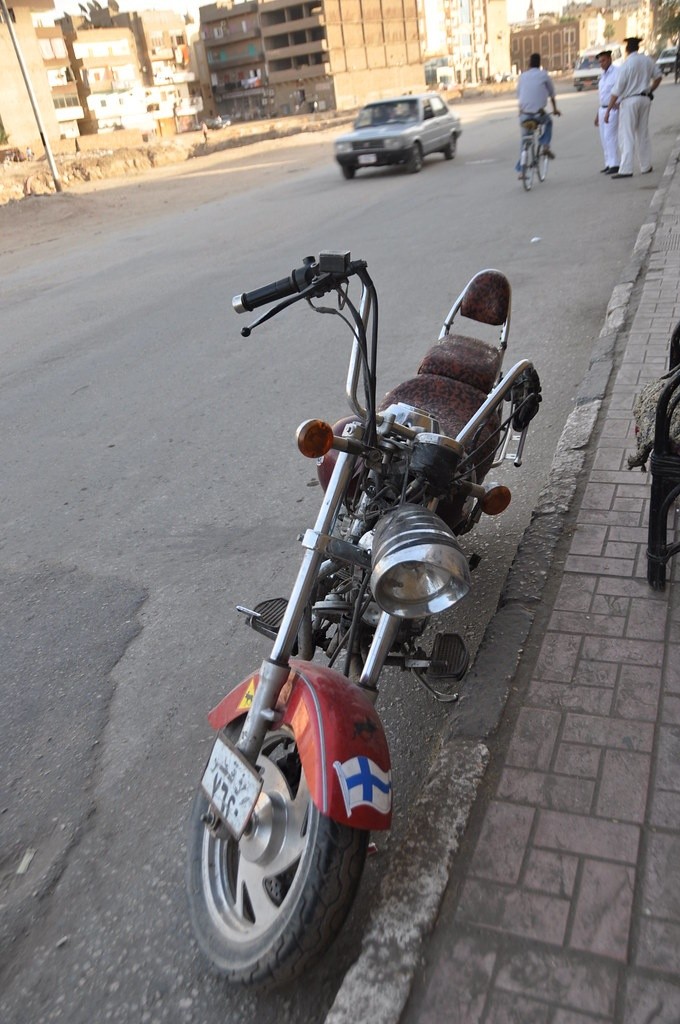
[178,249,542,998]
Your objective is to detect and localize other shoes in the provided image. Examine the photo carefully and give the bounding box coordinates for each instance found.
[611,173,633,178]
[542,144,555,160]
[599,166,609,172]
[641,165,653,174]
[606,166,619,174]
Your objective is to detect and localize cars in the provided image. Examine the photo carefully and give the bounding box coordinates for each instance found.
[334,92,462,180]
[191,114,233,129]
[656,46,678,74]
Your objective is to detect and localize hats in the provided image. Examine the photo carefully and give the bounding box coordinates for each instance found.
[624,37,641,45]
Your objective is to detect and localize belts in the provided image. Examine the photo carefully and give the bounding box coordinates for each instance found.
[640,93,650,97]
[601,106,608,108]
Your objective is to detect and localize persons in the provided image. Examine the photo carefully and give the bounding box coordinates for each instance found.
[27,146,33,161]
[595,51,623,174]
[14,150,26,162]
[604,36,663,179]
[202,123,207,141]
[516,53,561,171]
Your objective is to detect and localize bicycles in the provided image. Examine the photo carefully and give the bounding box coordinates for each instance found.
[517,111,561,191]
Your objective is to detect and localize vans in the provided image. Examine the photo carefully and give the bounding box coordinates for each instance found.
[572,45,622,93]
